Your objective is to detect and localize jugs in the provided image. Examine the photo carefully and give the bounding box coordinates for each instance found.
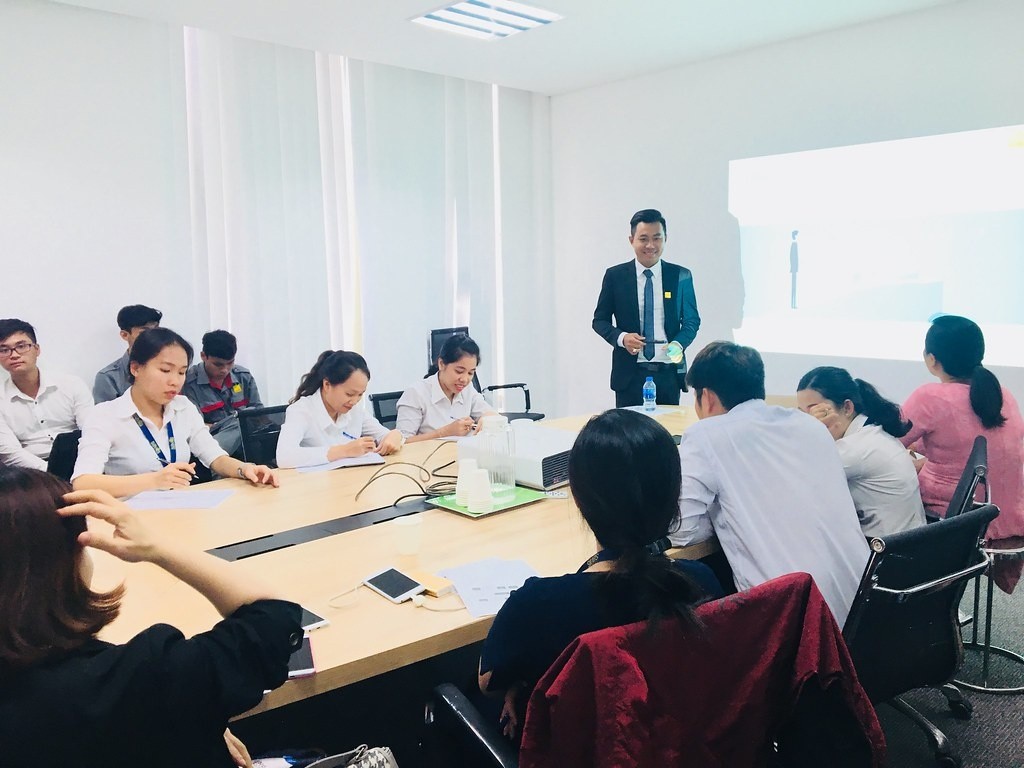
[477,416,516,504]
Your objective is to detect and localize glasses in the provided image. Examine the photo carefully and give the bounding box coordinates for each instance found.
[0,343,37,357]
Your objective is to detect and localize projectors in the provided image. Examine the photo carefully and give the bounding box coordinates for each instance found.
[455,422,578,492]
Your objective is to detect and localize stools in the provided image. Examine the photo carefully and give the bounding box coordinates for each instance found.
[955,537,1024,693]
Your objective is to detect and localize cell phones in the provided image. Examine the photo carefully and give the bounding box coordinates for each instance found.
[362,567,425,604]
[299,606,329,633]
[286,637,316,679]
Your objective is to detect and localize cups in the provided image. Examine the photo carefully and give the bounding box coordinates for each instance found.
[456,439,493,514]
[510,419,533,446]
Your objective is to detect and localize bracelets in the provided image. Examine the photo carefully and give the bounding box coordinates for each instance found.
[238,462,255,479]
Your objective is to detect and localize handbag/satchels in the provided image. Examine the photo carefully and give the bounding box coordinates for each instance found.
[305,744,399,768]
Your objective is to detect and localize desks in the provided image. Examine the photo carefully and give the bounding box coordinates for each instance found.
[88,405,721,727]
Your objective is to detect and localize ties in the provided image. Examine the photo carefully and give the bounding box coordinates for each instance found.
[642,269,655,361]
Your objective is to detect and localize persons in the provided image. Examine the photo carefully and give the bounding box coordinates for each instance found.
[478,409,718,742]
[591,209,701,411]
[0,465,305,768]
[395,334,498,445]
[667,341,871,633]
[276,350,406,470]
[797,367,927,544]
[93,305,163,405]
[0,318,96,474]
[897,316,1024,540]
[181,330,263,481]
[69,327,279,498]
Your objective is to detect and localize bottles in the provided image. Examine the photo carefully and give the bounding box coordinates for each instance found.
[642,376,656,416]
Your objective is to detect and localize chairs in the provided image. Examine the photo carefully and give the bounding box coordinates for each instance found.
[51,327,1000,768]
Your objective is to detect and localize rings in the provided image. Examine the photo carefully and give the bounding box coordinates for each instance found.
[824,409,828,416]
[633,349,636,352]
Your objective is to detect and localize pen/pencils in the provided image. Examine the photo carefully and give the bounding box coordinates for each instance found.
[450,416,475,431]
[642,340,667,344]
[342,432,357,440]
[158,459,200,479]
